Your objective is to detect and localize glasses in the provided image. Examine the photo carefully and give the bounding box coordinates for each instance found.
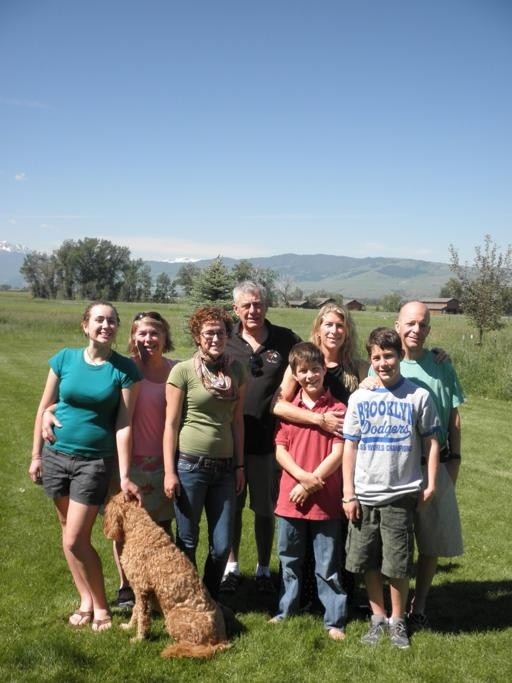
[134,312,162,320]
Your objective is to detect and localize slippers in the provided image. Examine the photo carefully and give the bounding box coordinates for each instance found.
[68,586,135,633]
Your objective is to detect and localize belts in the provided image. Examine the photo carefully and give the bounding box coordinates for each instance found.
[421,448,450,464]
[178,451,234,468]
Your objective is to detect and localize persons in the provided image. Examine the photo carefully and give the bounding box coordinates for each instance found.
[217,281,302,594]
[41,312,185,616]
[265,341,345,641]
[342,327,443,653]
[162,305,249,602]
[367,300,464,628]
[28,300,144,632]
[269,302,370,613]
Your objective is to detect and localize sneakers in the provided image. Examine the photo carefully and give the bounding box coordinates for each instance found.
[328,628,346,641]
[218,573,274,596]
[359,613,430,649]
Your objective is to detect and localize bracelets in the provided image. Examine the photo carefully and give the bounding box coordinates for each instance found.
[321,413,327,428]
[342,496,357,503]
[234,465,244,470]
[42,410,53,416]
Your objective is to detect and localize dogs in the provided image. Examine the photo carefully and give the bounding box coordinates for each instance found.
[103,488,237,663]
